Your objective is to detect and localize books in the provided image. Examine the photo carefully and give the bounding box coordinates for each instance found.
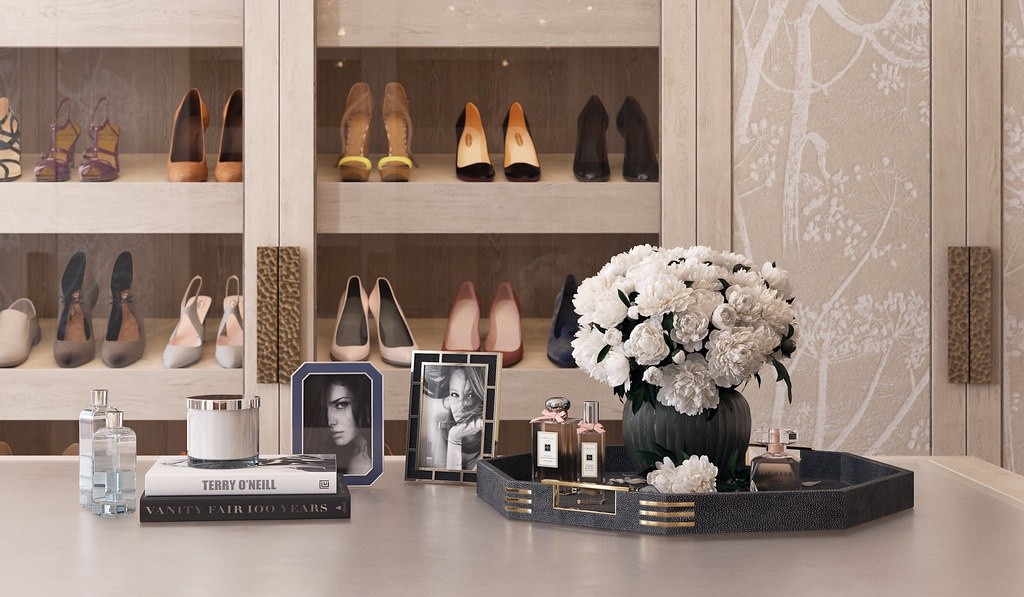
[140,453,352,522]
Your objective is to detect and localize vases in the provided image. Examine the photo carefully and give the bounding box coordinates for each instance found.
[618,387,752,479]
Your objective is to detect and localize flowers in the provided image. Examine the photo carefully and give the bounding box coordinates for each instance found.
[570,244,799,415]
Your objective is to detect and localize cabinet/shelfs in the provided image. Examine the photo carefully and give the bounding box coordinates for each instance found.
[314,152,666,418]
[0,154,247,422]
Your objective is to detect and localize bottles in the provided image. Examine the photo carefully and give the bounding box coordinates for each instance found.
[78,389,137,517]
[531,397,607,487]
[746,426,803,491]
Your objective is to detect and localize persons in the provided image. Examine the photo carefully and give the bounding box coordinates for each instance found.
[318,374,372,474]
[430,367,483,470]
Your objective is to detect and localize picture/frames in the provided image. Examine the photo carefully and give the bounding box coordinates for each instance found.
[403,349,503,486]
[290,361,386,487]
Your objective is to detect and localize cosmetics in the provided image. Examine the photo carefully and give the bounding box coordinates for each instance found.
[79,390,136,517]
[745,428,798,492]
[530,397,606,484]
[187,395,261,466]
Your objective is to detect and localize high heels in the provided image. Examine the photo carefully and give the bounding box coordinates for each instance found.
[0,77,663,372]
